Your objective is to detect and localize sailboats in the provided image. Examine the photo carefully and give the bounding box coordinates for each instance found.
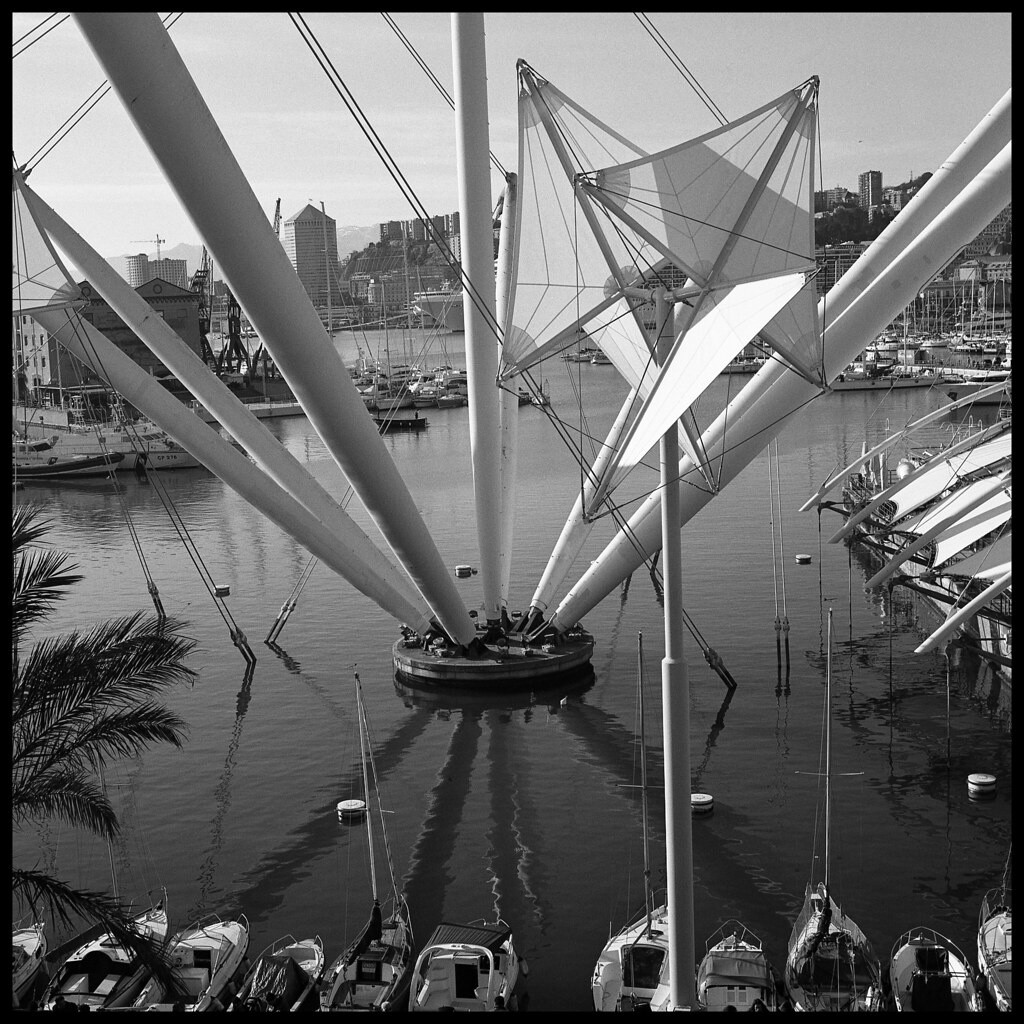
[34,643,176,1013]
[316,668,416,1012]
[589,630,681,1012]
[784,605,887,1011]
[330,284,553,413]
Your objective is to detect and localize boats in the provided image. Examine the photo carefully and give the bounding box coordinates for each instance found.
[217,932,328,1012]
[11,422,234,484]
[697,916,776,1011]
[889,888,1012,1010]
[11,905,51,1013]
[131,909,255,1011]
[560,328,1013,406]
[408,912,524,1013]
